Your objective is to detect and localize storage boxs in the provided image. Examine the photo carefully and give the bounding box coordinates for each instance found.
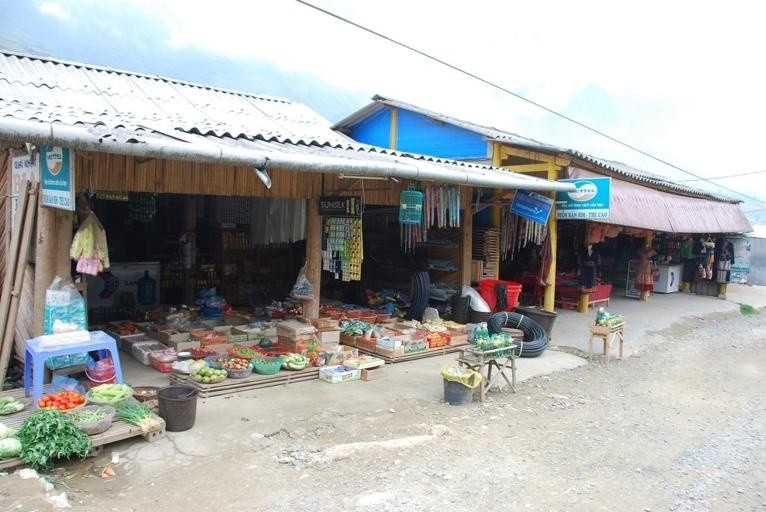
[339,309,468,358]
[111,301,385,384]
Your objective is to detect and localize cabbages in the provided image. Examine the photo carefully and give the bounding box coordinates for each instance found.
[0,423,22,459]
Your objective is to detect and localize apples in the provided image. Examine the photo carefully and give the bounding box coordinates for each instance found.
[191,358,249,383]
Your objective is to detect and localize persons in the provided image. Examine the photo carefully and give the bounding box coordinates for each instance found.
[635,243,656,289]
[681,235,697,283]
[578,244,602,291]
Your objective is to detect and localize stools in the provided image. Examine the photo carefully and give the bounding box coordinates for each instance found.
[23,328,124,413]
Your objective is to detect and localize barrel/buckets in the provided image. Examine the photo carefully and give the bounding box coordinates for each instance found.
[157,385,198,432]
[480,279,523,313]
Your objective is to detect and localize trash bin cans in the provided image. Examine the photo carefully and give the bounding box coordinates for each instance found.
[730,264,750,284]
[440,363,482,406]
[515,307,557,336]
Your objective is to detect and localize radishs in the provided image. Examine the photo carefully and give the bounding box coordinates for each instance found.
[279,353,310,370]
[2,396,25,412]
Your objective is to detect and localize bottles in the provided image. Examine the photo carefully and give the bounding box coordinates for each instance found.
[477,333,512,357]
[466,323,487,342]
[595,306,622,327]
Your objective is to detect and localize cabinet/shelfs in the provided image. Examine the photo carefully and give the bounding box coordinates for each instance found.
[588,307,626,361]
[454,332,518,402]
[625,259,654,299]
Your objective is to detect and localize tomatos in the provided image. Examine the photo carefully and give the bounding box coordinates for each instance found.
[38,391,85,410]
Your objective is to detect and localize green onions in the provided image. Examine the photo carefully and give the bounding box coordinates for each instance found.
[112,398,160,434]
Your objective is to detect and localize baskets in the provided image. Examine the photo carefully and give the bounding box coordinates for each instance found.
[131,386,161,402]
[319,365,361,384]
[332,309,392,324]
[148,348,178,373]
[427,332,451,348]
